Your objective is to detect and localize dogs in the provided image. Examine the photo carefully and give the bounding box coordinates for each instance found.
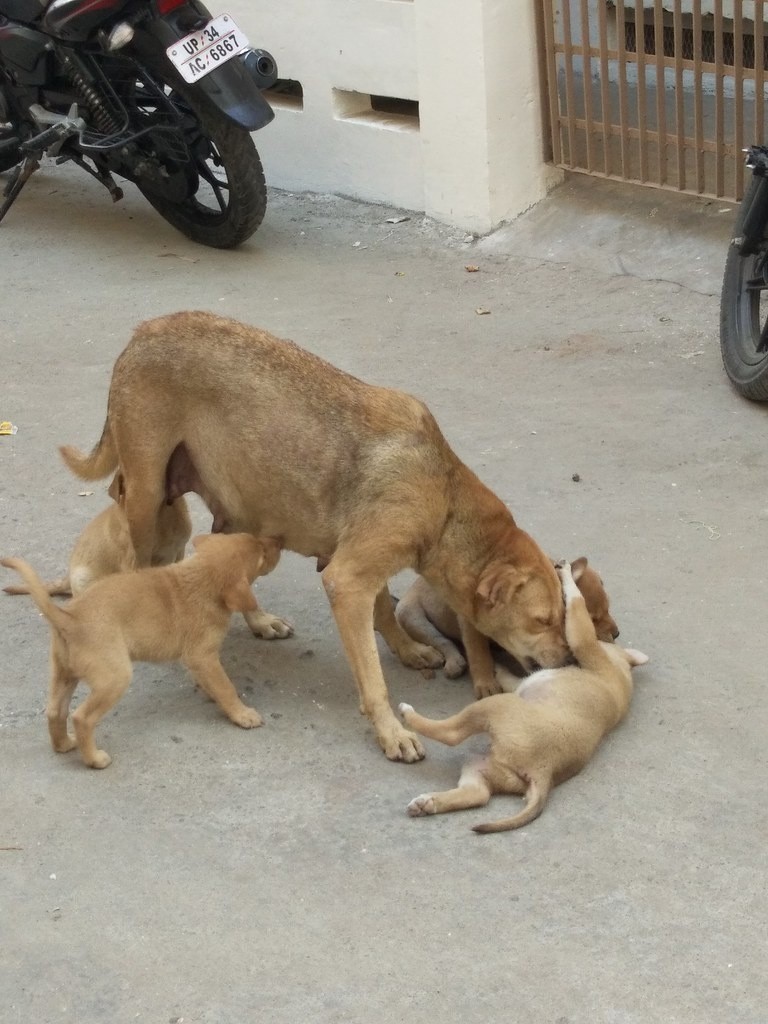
[0,309,650,835]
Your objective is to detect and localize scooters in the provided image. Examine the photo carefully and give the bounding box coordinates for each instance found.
[719,140,768,403]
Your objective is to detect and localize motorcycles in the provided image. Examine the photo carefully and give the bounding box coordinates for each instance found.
[0,0,280,250]
